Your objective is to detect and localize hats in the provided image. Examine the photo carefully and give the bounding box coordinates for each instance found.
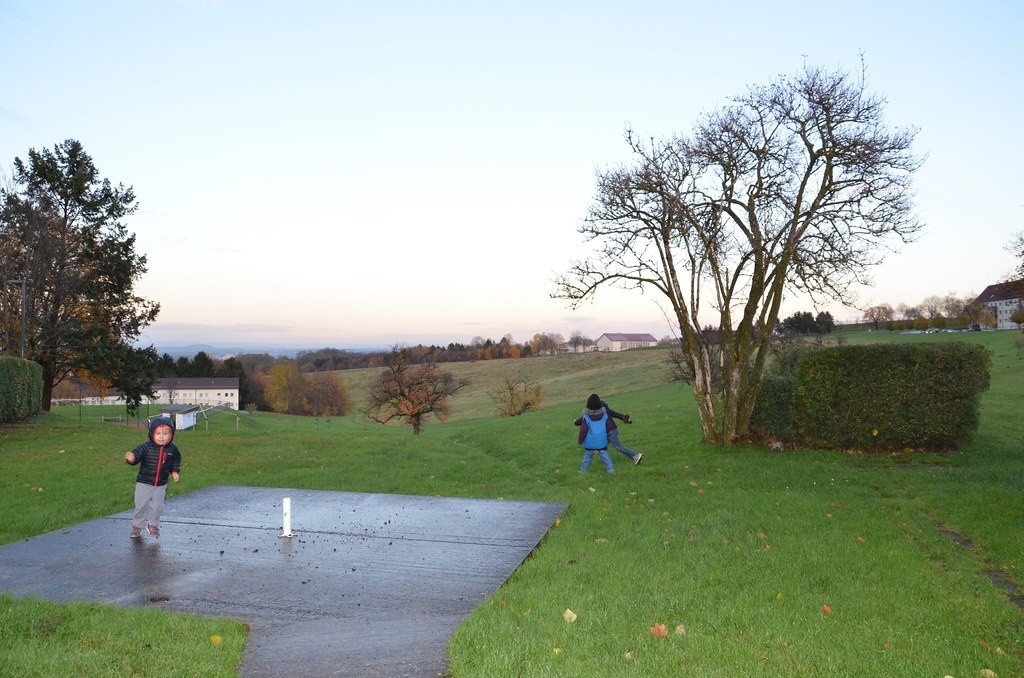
[586,394,602,410]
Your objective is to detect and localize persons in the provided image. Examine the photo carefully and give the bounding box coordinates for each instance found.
[573,393,644,474]
[123,416,182,540]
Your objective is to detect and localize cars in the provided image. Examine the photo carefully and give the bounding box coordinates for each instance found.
[925,325,994,335]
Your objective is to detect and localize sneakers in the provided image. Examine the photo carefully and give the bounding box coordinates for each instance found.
[633,453,643,465]
[130,526,143,537]
[147,524,160,537]
